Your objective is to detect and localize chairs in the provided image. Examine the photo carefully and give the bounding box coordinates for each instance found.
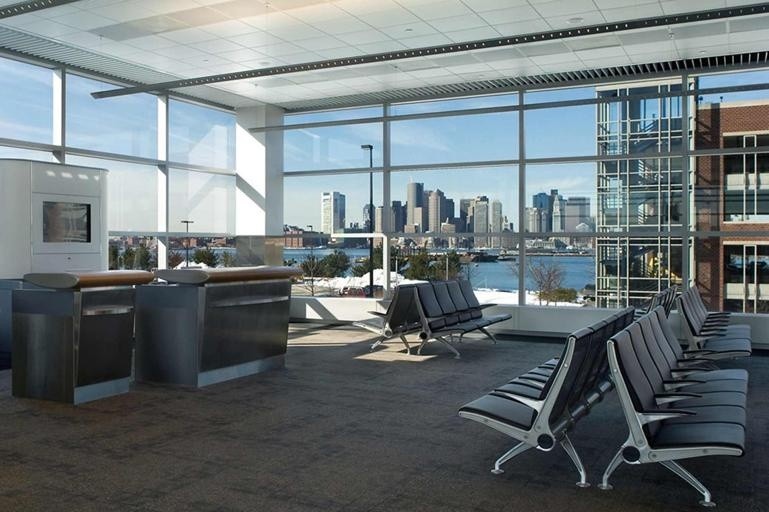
[457,285,752,506]
[352,279,513,359]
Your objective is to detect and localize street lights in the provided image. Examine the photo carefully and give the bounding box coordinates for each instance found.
[182,221,194,267]
[361,144,374,299]
[306,224,313,255]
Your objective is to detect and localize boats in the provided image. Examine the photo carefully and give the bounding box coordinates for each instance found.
[458,251,517,263]
